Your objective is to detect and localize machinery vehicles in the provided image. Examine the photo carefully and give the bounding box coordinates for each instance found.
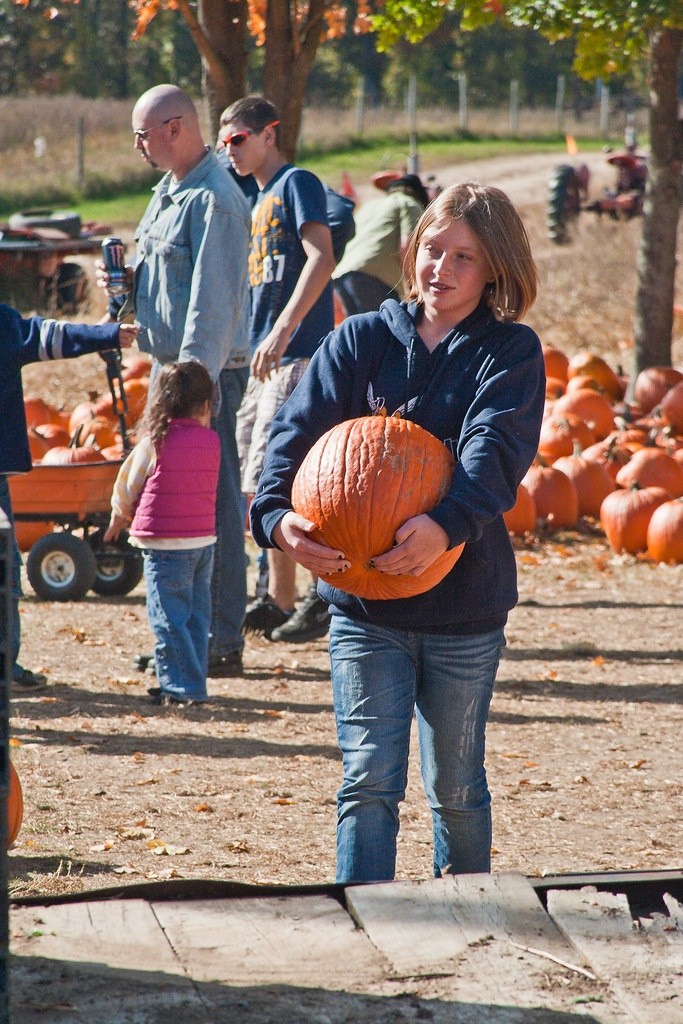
[548,141,649,246]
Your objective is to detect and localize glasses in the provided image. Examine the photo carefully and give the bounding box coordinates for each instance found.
[222,121,280,147]
[135,116,182,141]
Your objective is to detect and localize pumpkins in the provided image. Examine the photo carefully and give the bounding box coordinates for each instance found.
[10,520,54,552]
[503,342,683,566]
[8,759,24,848]
[23,357,149,462]
[291,416,466,600]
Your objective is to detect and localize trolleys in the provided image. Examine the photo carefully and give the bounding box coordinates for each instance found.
[2,348,143,602]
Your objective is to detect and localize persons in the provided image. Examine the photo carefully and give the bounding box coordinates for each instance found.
[214,94,355,642]
[0,303,140,692]
[249,182,547,883]
[94,84,252,678]
[331,173,430,319]
[104,361,221,706]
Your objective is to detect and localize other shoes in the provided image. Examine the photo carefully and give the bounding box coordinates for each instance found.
[271,581,331,642]
[147,687,207,706]
[132,651,243,675]
[241,597,297,639]
[10,669,47,692]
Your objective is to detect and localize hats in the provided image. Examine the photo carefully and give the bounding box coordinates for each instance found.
[393,174,428,206]
